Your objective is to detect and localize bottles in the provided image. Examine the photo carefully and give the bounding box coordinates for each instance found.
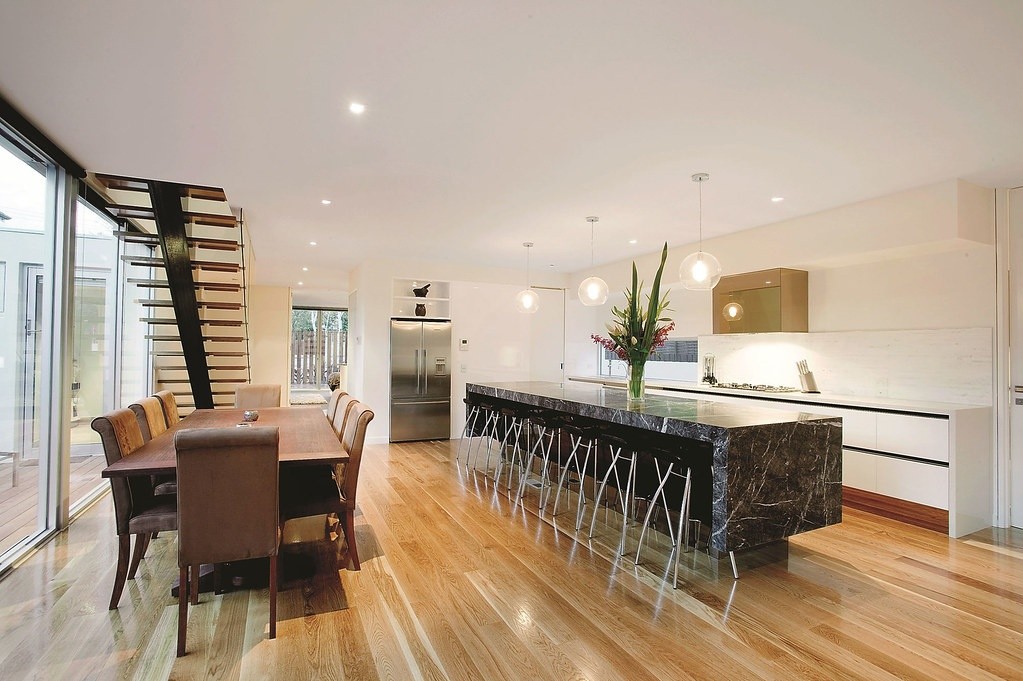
[415,304,426,316]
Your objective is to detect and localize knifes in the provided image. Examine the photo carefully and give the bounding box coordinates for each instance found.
[795,359,809,375]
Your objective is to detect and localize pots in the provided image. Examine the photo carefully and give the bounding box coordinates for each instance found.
[413,284,430,296]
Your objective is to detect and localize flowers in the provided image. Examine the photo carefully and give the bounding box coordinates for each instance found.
[591,241,675,398]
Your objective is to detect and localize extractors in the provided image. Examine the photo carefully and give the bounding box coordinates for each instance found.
[713,268,809,334]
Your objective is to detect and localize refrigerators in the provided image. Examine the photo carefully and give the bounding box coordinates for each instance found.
[390,319,452,443]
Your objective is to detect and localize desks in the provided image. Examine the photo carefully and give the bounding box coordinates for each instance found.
[101,405,350,598]
[466,380,843,561]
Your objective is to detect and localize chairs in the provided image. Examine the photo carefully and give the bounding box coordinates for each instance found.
[174,426,284,657]
[280,389,374,570]
[235,384,281,409]
[92,390,177,609]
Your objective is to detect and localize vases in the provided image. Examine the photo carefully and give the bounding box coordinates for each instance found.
[624,356,648,402]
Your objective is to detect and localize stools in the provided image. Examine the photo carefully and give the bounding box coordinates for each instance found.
[456,398,739,590]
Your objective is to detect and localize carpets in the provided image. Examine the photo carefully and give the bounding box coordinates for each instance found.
[20,454,91,467]
[288,393,328,405]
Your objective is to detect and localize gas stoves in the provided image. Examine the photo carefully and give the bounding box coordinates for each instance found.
[712,383,801,393]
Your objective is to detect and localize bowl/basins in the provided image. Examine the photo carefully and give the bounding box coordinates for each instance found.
[244,410,259,421]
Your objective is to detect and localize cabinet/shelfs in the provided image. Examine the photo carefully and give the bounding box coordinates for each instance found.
[391,276,452,318]
[712,266,808,333]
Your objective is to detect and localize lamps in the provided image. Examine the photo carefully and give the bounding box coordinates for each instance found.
[577,216,609,307]
[517,242,540,313]
[722,292,743,321]
[680,173,721,290]
